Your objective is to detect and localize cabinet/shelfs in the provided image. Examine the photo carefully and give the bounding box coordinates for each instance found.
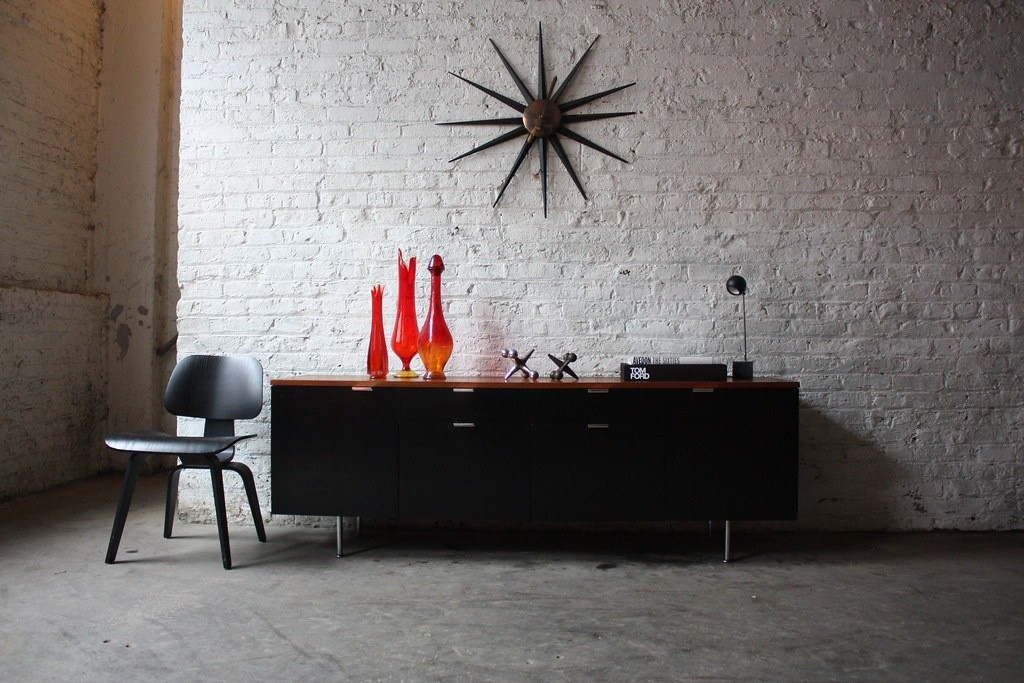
[269,373,799,561]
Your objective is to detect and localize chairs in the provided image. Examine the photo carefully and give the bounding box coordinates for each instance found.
[106,354,266,571]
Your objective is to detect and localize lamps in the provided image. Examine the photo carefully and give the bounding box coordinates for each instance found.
[726,274,753,378]
